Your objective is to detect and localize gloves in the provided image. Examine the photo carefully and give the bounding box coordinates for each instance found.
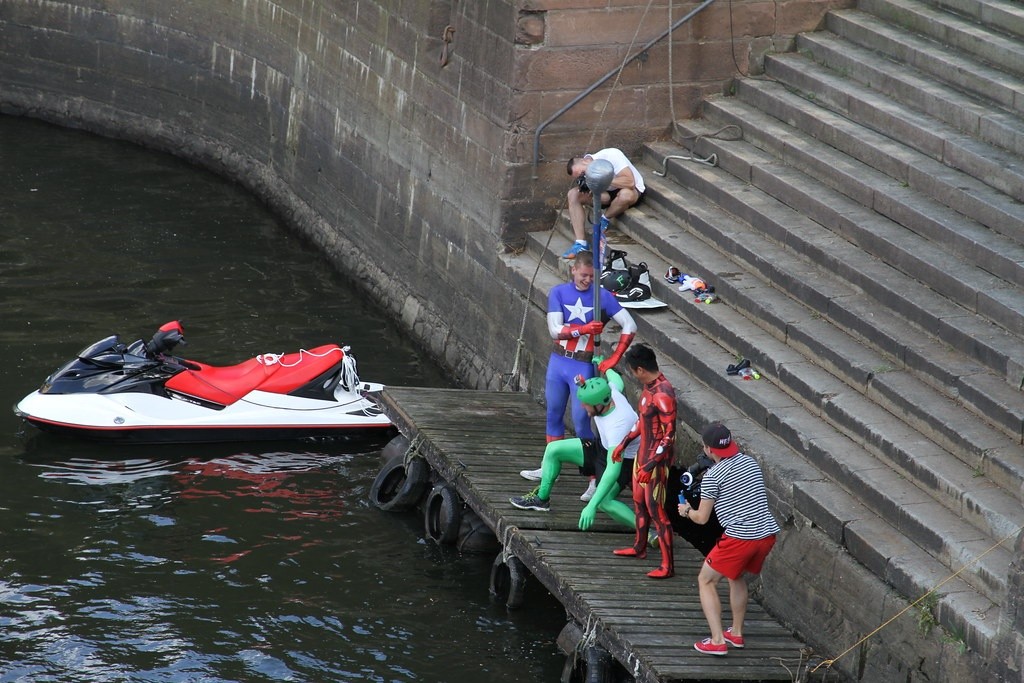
[678,275,705,290]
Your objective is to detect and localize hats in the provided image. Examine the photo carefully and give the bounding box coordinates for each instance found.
[702,423,738,457]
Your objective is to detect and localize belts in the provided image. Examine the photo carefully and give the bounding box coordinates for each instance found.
[552,342,594,361]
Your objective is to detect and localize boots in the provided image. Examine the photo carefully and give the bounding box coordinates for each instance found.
[615,264,653,302]
[599,249,627,284]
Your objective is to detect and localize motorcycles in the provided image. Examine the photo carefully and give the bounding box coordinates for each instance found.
[13,320,402,441]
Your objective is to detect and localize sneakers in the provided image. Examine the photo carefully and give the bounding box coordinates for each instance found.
[520,468,560,482]
[723,627,744,647]
[600,216,609,233]
[562,241,589,258]
[580,479,596,501]
[646,528,659,549]
[694,638,728,654]
[509,486,551,512]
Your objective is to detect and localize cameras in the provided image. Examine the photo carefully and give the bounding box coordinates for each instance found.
[577,173,592,193]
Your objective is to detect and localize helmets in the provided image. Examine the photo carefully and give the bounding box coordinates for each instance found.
[604,268,630,291]
[577,377,612,406]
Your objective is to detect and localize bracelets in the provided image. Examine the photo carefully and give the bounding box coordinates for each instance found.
[685,507,692,516]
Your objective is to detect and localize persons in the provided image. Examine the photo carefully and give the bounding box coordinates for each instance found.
[611,342,677,579]
[520,250,639,501]
[676,423,780,655]
[509,353,658,549]
[561,147,647,258]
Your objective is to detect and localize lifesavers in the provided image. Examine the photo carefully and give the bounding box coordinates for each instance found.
[368,453,429,512]
[560,643,613,683]
[489,550,524,606]
[424,485,460,545]
